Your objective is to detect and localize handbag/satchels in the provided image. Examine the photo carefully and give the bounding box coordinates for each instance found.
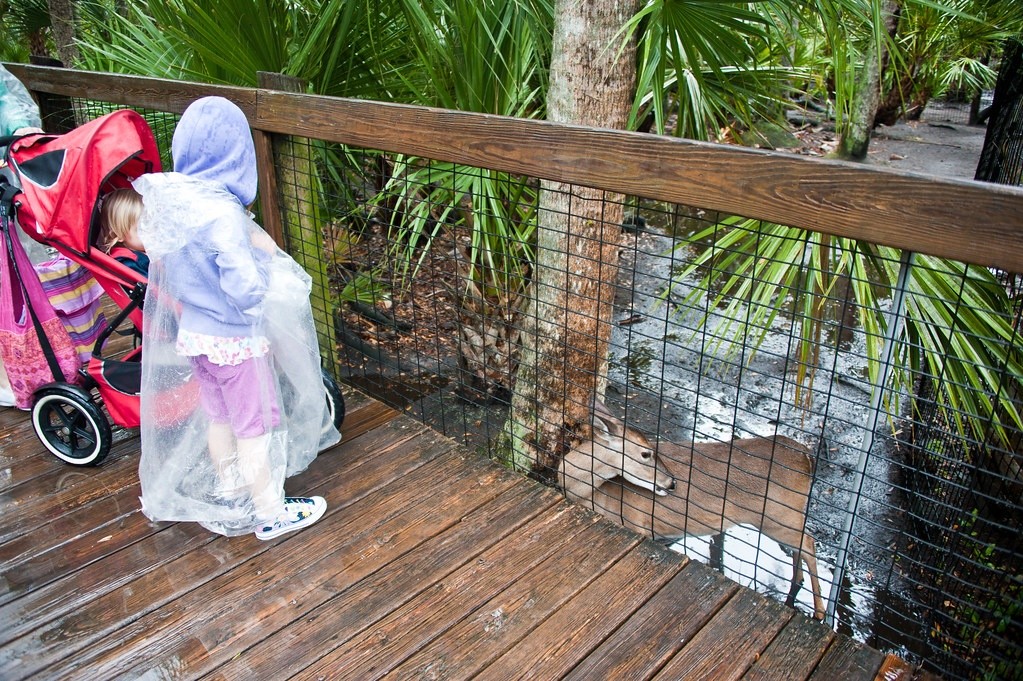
[22,254,109,362]
[0,214,83,413]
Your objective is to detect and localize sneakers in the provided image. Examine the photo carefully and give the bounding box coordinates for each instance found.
[256,494,328,540]
[211,488,255,533]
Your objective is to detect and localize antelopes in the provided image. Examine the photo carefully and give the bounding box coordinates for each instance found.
[557,395,827,625]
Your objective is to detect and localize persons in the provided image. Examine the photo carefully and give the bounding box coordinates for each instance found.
[0,61,46,167]
[163,96,328,541]
[101,188,150,279]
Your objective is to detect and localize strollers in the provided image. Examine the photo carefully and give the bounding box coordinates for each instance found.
[0,110,346,468]
